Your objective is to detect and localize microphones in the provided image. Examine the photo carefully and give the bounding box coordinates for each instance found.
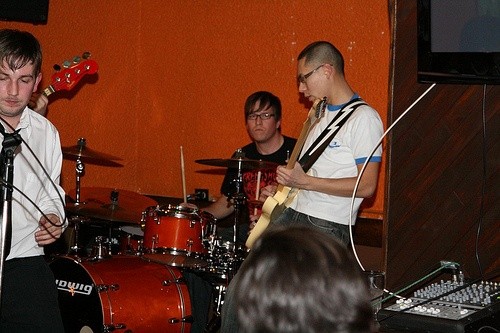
[187,189,212,198]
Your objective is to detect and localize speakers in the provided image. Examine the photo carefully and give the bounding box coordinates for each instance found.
[0,0,48,25]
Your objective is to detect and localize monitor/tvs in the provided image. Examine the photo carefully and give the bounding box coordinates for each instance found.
[415,0,500,86]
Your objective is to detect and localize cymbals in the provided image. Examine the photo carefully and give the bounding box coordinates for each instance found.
[59,145,118,162]
[69,187,160,222]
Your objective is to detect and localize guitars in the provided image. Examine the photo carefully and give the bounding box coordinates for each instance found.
[194,156,281,170]
[40,51,101,97]
[245,95,328,250]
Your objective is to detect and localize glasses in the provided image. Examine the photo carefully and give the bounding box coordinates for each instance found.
[298,64,333,82]
[248,113,274,120]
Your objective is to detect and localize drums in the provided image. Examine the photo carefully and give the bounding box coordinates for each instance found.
[91,235,119,260]
[48,254,192,333]
[141,205,216,270]
[204,245,249,272]
[118,226,145,256]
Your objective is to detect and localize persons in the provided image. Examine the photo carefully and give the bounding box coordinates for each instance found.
[179,90,302,333]
[234,225,378,333]
[259,40,384,247]
[0,30,69,333]
